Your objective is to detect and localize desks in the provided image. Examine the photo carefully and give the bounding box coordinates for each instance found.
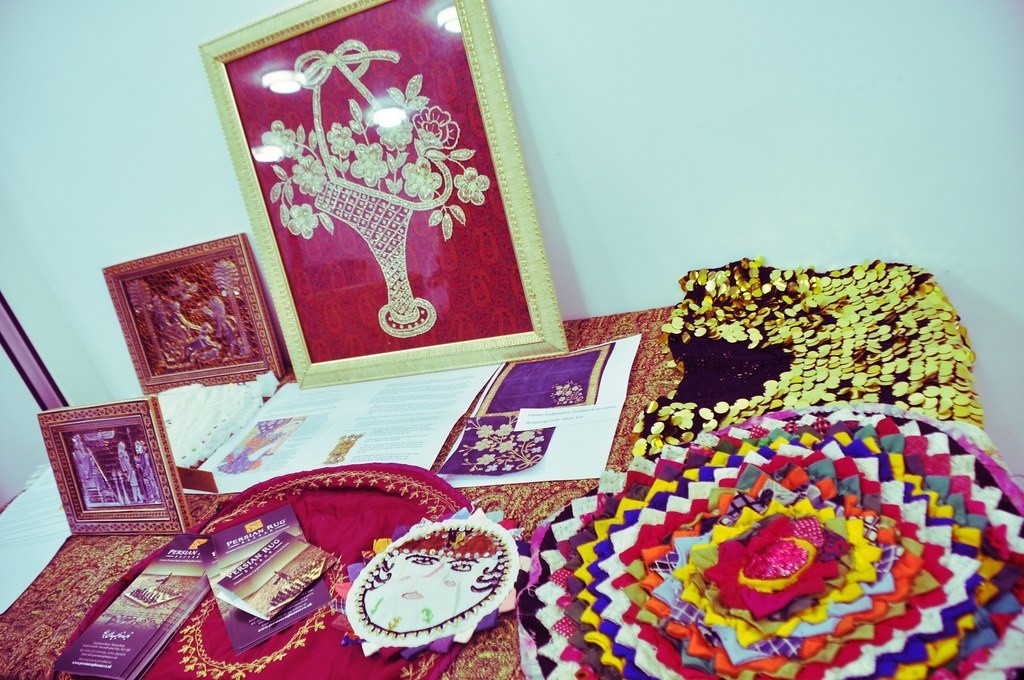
[0,264,987,680]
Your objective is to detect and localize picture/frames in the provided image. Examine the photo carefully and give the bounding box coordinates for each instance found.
[36,396,192,535]
[102,233,286,396]
[199,0,569,390]
